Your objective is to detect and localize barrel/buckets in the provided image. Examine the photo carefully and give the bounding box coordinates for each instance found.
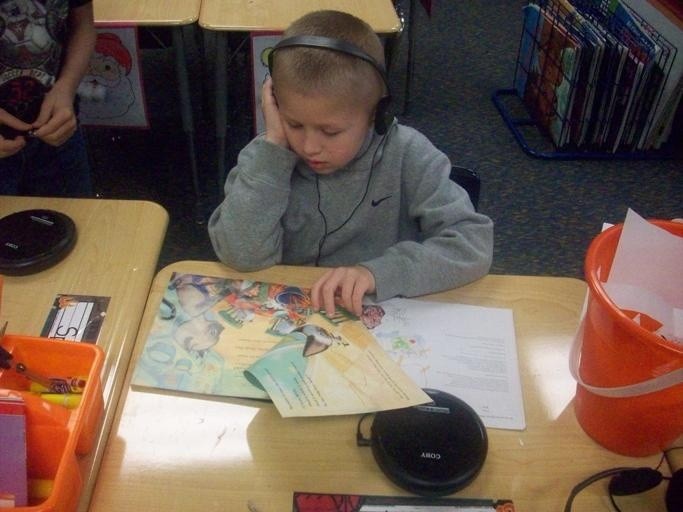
[568,217,683,458]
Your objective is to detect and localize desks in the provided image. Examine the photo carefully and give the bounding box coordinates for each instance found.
[87,260,672,512]
[0,196,169,512]
[198,0,402,197]
[74,0,204,225]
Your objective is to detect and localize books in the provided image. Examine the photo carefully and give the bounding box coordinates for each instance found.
[130,271,526,431]
[512,0,683,151]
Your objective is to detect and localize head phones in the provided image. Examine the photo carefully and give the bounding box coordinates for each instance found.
[267,35,395,135]
[564,467,683,512]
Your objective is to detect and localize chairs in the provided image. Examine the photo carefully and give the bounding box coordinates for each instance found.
[224,143,481,213]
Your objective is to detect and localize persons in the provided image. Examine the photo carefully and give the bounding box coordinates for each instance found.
[0,0,97,197]
[206,11,494,319]
